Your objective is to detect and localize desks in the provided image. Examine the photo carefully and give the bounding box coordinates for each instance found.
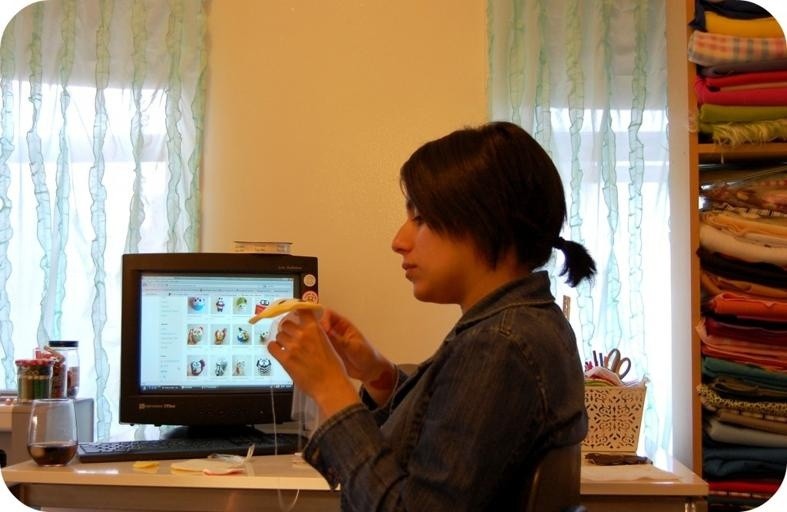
[1,442,713,511]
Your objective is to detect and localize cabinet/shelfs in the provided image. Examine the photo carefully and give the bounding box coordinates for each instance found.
[669,0,787,511]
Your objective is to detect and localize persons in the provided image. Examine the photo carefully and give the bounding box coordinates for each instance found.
[267,122,598,512]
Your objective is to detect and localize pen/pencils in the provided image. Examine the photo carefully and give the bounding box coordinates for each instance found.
[583,350,607,372]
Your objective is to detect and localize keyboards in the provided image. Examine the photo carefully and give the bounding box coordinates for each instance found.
[76,433,308,463]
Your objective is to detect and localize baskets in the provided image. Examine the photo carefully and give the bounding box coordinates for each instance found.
[582,386,646,452]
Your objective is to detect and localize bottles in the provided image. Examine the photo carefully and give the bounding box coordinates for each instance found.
[17,340,81,398]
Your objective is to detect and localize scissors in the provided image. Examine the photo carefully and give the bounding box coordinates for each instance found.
[605,348,632,380]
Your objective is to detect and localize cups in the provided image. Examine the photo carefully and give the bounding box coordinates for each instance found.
[26,397,78,467]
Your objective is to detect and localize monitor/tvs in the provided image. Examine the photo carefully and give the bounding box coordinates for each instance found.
[119,253,318,438]
